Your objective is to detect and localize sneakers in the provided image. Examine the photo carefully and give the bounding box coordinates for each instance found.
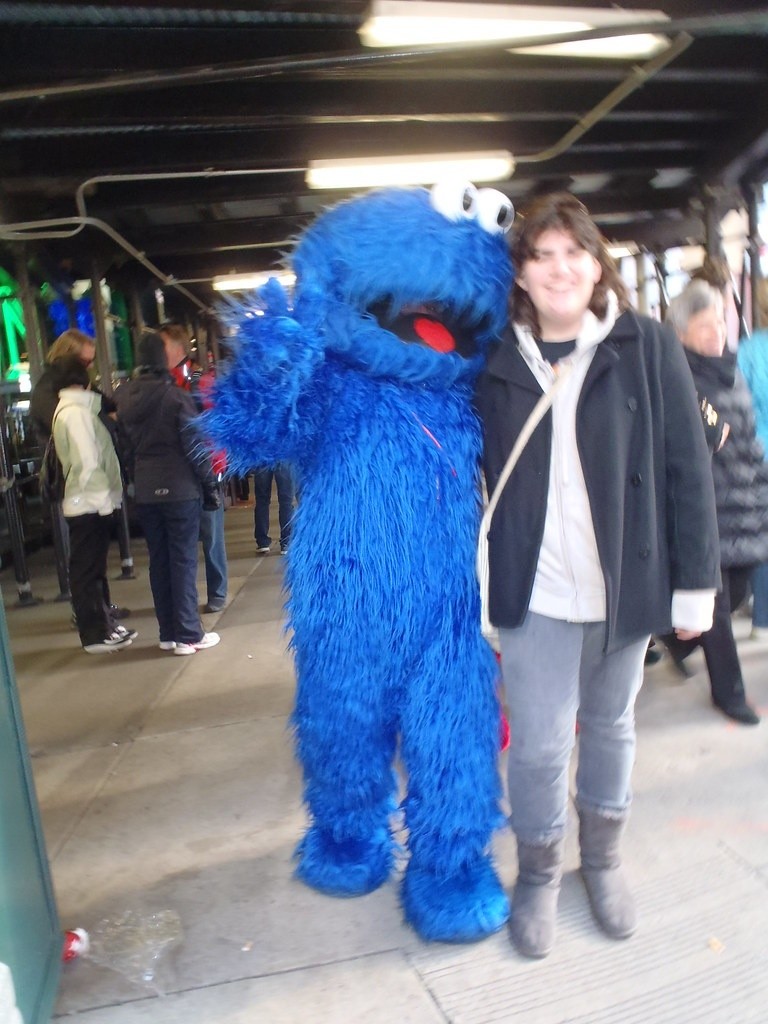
[114,623,139,639]
[174,632,221,655]
[84,632,132,654]
[159,641,176,650]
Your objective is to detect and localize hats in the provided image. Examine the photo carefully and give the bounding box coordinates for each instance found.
[137,332,165,366]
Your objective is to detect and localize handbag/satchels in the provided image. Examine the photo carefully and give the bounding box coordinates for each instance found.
[475,512,501,654]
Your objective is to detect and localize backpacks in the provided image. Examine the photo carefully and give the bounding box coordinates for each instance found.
[38,403,72,500]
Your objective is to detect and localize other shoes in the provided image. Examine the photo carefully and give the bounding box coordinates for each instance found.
[644,650,661,665]
[255,542,269,552]
[203,602,225,612]
[658,632,693,676]
[711,700,760,724]
[109,604,130,619]
[280,544,288,554]
[749,624,768,639]
[69,611,79,629]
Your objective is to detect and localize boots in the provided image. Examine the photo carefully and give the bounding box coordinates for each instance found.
[508,829,567,959]
[573,789,639,939]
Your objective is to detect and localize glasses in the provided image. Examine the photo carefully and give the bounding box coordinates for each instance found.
[81,357,96,366]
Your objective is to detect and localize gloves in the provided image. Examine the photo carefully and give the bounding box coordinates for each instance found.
[202,485,221,511]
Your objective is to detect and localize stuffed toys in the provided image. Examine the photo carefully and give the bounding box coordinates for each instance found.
[186,173,514,946]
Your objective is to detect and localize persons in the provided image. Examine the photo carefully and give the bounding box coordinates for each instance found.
[638,279,768,727]
[27,329,297,654]
[471,193,724,957]
